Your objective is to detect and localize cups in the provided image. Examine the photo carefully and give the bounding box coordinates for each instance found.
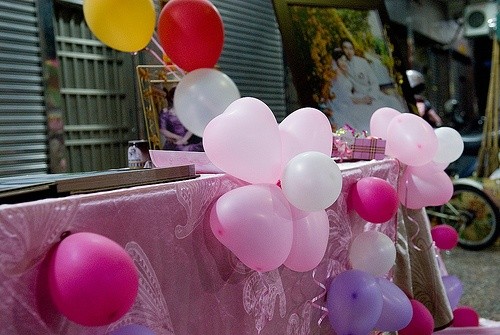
[127,140,151,169]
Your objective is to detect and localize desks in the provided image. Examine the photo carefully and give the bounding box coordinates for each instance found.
[0,159,454,335]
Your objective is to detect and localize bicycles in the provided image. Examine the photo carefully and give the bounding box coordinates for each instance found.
[425,178,500,251]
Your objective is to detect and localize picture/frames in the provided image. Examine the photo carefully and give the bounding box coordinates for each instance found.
[270,0,421,136]
[136,64,219,153]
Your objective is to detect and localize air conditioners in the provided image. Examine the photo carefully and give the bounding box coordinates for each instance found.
[465,2,497,35]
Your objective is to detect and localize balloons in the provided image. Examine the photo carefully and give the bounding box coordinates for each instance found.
[174,67,240,137]
[431,225,458,249]
[83,0,156,52]
[210,183,293,272]
[327,270,383,335]
[277,108,332,175]
[203,98,282,184]
[350,232,396,274]
[398,299,434,335]
[351,108,464,223]
[158,0,224,71]
[374,278,413,331]
[452,307,478,327]
[282,203,330,272]
[48,232,139,327]
[442,275,463,307]
[282,152,343,210]
[110,325,154,335]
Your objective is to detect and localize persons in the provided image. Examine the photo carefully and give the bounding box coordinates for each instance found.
[408,70,482,176]
[160,87,204,153]
[327,41,403,134]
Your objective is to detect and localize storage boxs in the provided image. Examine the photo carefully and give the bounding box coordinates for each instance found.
[332,131,388,160]
